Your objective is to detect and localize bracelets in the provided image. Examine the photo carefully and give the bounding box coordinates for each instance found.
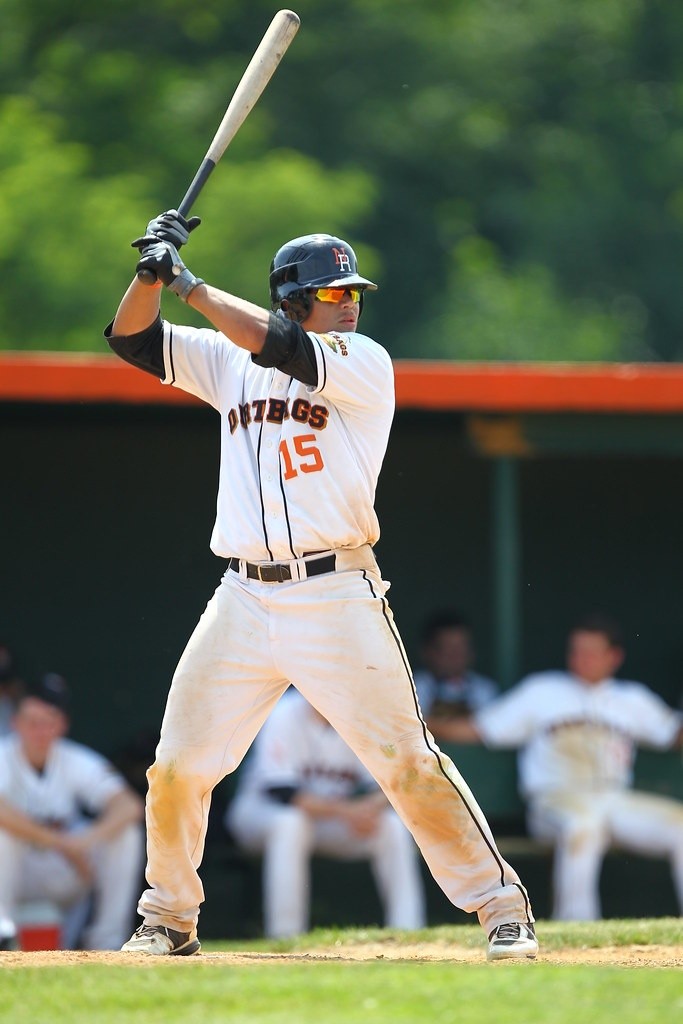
[181,278,204,303]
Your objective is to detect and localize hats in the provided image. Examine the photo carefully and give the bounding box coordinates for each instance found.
[270,234,378,303]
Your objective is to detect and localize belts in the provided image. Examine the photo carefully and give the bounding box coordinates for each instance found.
[229,554,336,582]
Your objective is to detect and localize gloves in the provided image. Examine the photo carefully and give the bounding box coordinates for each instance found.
[131,235,206,305]
[145,209,201,252]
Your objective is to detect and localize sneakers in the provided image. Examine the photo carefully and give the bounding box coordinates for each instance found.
[120,925,201,955]
[487,923,539,959]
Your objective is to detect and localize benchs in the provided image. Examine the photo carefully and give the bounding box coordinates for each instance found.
[431,742,683,856]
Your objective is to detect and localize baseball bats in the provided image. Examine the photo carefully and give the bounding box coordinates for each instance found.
[135,8,301,285]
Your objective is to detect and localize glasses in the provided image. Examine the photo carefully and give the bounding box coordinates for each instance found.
[311,288,363,303]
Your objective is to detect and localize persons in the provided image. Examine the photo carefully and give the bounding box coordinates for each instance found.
[413,615,514,829]
[104,209,538,959]
[426,621,683,920]
[0,690,146,952]
[228,690,425,938]
